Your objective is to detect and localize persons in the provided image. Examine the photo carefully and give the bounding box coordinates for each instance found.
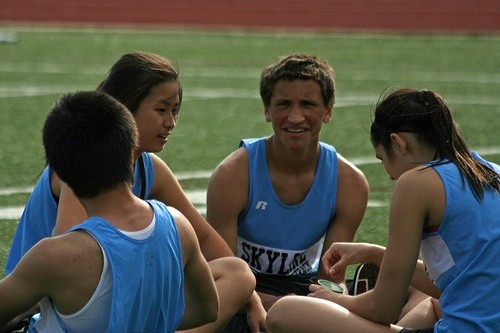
[0,90,220,333]
[205,52,370,333]
[264,86,500,333]
[4,49,267,333]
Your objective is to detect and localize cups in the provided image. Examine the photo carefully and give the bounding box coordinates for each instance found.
[318,280,344,294]
